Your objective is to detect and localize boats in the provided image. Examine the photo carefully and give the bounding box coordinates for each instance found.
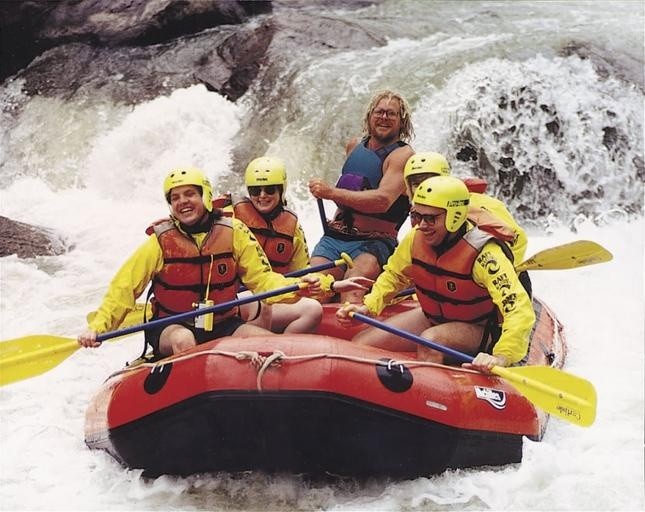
[84,297,563,472]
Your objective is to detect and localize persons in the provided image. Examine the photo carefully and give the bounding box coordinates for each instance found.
[403,150,534,307]
[212,153,376,306]
[77,165,324,356]
[334,174,536,376]
[310,86,414,305]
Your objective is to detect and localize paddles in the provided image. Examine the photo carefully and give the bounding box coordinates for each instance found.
[86,252,353,341]
[348,312,596,428]
[395,241,612,297]
[0,282,310,389]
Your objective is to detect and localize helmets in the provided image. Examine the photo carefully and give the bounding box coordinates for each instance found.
[404,152,450,199]
[244,156,286,205]
[413,175,470,233]
[163,167,212,214]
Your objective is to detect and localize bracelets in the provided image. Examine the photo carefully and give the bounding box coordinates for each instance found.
[329,279,338,294]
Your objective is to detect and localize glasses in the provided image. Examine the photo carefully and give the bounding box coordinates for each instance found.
[372,109,401,119]
[247,185,275,196]
[414,213,447,226]
